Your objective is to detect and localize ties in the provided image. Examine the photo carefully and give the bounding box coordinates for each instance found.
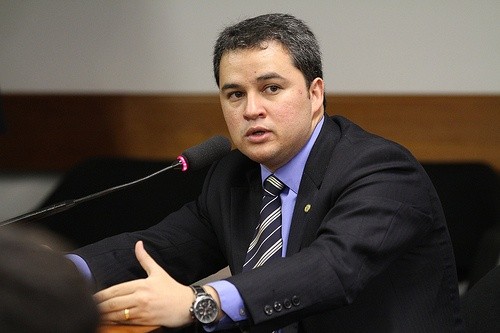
[241,175,286,270]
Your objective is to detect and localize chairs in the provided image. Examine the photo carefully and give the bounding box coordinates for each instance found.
[10,158,499,301]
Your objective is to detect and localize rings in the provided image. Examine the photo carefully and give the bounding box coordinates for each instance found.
[125,309,130,319]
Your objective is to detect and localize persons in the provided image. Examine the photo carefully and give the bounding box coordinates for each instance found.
[66,14,460,333]
[0,239,99,333]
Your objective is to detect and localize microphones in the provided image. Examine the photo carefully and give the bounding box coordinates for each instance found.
[0,136,232,228]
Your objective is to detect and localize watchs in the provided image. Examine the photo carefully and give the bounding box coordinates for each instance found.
[189,284,219,328]
